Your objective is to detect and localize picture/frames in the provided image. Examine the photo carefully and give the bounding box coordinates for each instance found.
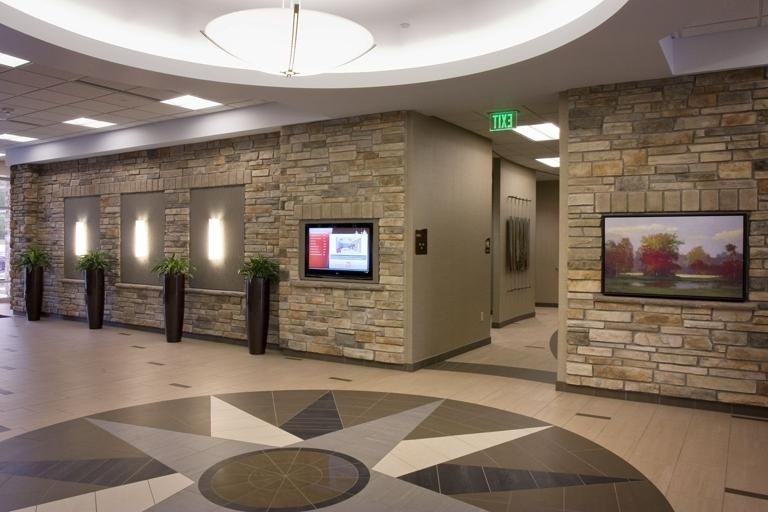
[599,208,750,302]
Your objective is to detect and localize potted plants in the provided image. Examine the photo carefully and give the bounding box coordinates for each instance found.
[152,255,195,342]
[16,243,51,321]
[72,246,114,328]
[238,254,280,353]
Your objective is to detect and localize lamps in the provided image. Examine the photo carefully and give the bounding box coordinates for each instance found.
[201,0,376,77]
[206,218,222,263]
[75,220,87,257]
[133,219,146,259]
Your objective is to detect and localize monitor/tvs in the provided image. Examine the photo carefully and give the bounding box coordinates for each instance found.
[302,221,372,276]
